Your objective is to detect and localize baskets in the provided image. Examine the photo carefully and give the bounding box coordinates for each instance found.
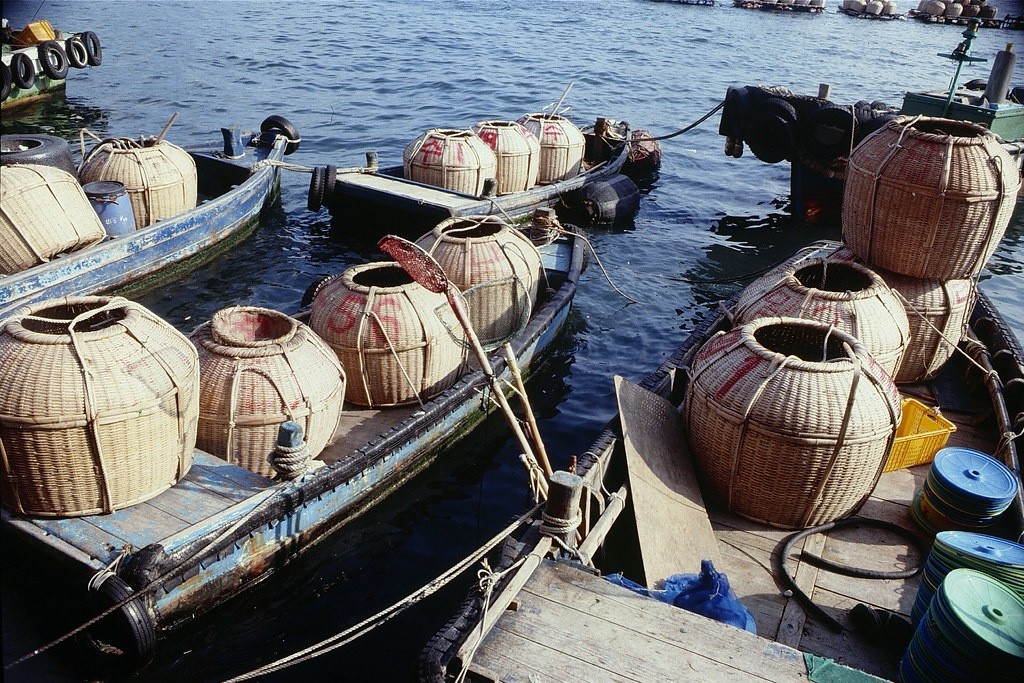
[880,395,957,473]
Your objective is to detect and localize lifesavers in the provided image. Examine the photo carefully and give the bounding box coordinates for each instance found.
[38,41,69,80]
[260,115,300,155]
[73,572,157,669]
[716,84,749,139]
[744,98,800,164]
[80,31,103,66]
[307,164,337,213]
[0,60,12,102]
[66,37,88,68]
[965,78,1024,105]
[11,53,35,89]
[801,105,860,158]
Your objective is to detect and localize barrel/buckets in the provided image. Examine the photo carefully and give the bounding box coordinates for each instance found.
[81,180,136,242]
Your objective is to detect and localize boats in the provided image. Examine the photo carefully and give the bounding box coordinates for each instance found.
[418,235,1024,683]
[718,39,1024,230]
[0,207,593,683]
[332,117,632,239]
[0,17,103,112]
[0,112,302,340]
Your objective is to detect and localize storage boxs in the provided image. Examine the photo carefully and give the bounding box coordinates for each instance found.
[881,398,957,473]
[13,21,55,49]
